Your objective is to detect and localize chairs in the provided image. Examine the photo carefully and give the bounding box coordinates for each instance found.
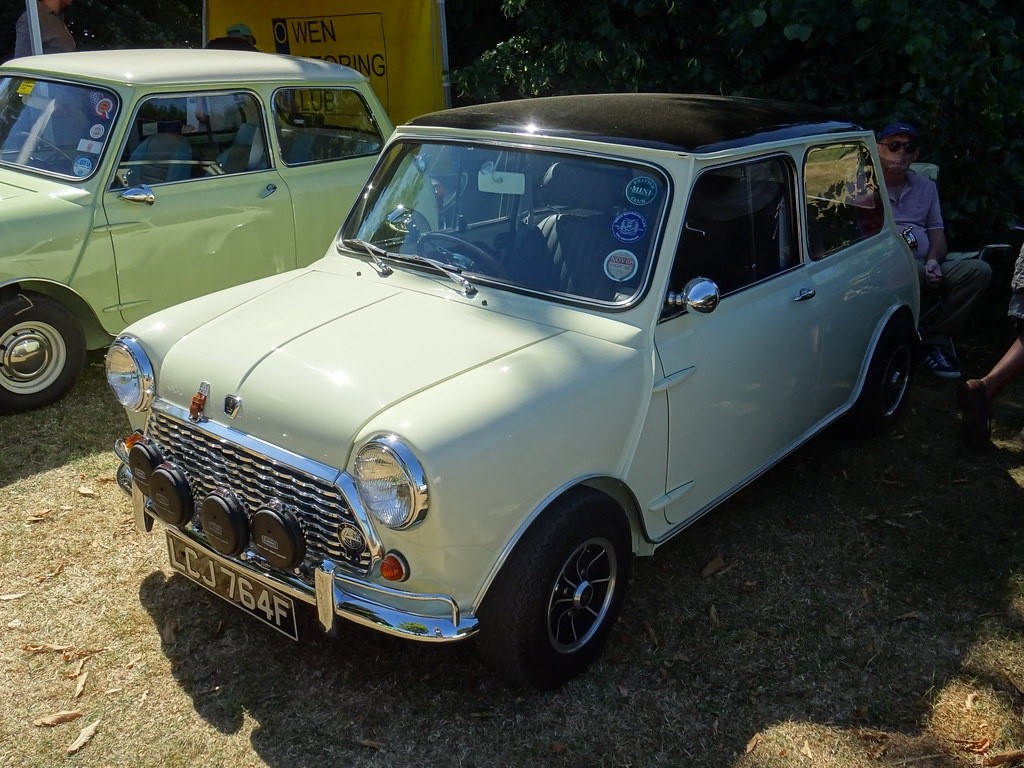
[681,163,796,283]
[507,212,634,307]
[116,133,272,189]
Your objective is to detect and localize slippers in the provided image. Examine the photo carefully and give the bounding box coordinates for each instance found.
[956,382,992,450]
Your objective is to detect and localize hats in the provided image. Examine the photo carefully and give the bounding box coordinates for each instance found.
[226,23,257,45]
[876,122,919,144]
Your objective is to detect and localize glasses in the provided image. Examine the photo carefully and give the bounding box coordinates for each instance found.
[876,140,918,154]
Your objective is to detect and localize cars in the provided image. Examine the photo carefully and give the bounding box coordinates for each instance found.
[105,93,924,693]
[0,48,444,415]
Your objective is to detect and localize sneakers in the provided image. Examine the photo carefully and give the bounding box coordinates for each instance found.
[919,344,961,378]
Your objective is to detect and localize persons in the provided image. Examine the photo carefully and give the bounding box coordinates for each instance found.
[954,242,1024,448]
[14,0,77,58]
[196,22,260,133]
[850,122,994,380]
